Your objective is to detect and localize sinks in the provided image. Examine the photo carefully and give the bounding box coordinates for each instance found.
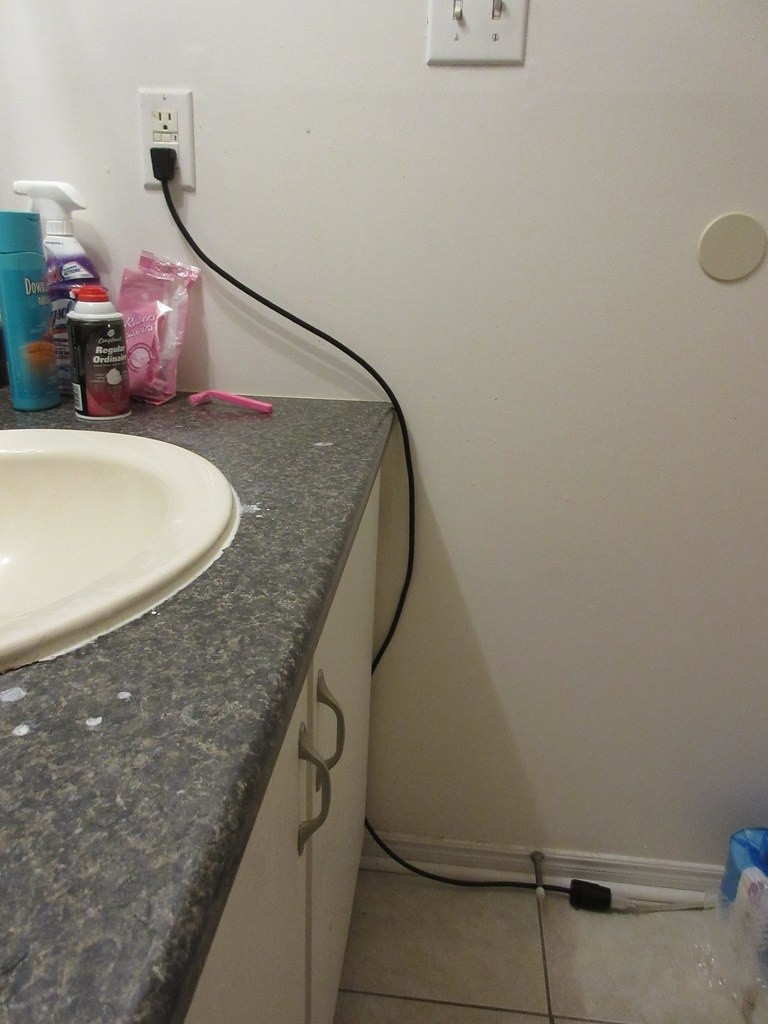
[0,432,241,667]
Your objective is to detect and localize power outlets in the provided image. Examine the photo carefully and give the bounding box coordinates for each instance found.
[140,88,194,190]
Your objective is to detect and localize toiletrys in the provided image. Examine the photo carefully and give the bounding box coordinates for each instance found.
[0,212,62,409]
[12,181,104,394]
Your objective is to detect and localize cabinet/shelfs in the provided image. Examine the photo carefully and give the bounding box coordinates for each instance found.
[183,468,381,1024]
